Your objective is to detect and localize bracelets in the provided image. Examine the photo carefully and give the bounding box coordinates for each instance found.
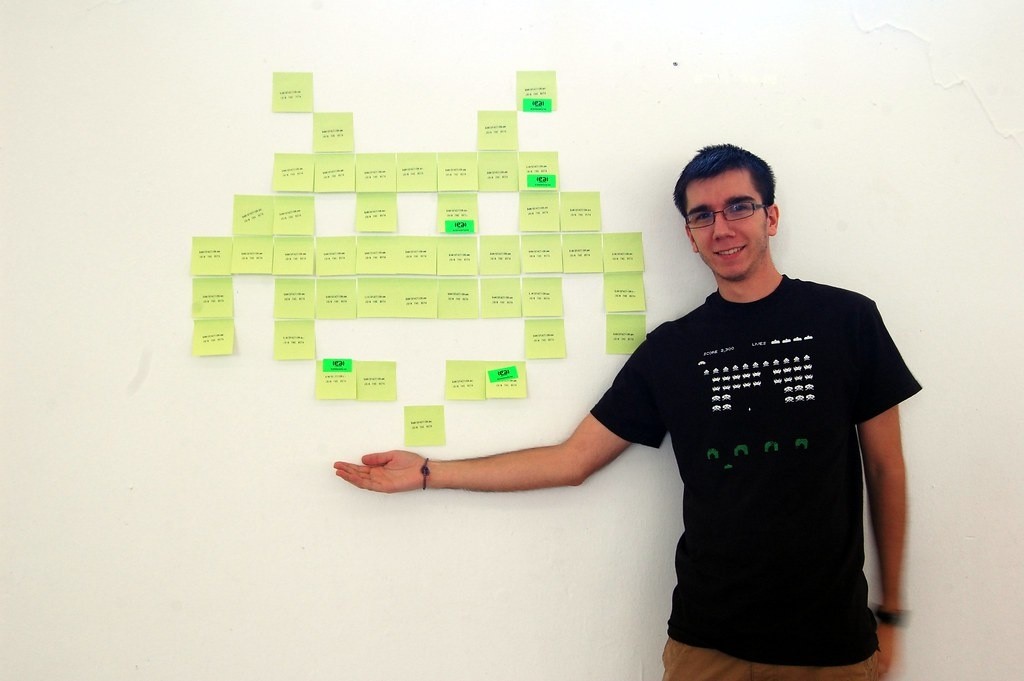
[875,606,905,624]
[422,458,430,489]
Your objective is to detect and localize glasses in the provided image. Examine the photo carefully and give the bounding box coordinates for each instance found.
[686,202,768,228]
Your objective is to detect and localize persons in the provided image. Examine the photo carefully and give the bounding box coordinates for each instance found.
[333,143,922,680]
